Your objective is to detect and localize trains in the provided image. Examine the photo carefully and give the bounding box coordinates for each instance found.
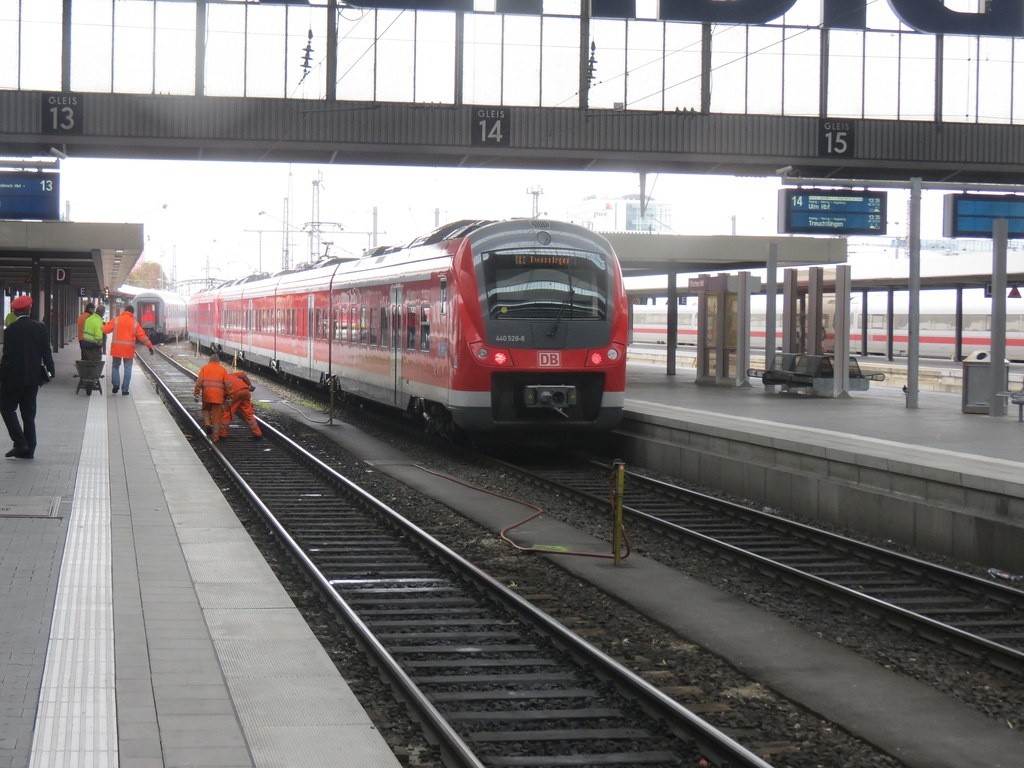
[131,291,187,343]
[184,218,629,445]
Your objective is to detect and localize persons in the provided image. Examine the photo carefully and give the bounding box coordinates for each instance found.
[84,305,107,364]
[0,295,56,459]
[102,306,155,396]
[193,354,234,443]
[218,370,263,441]
[5,309,18,329]
[77,302,95,360]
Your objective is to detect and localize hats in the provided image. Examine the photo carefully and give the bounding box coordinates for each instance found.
[13,297,31,311]
[209,354,220,362]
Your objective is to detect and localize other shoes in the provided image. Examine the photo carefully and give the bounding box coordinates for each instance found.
[6,446,34,460]
[112,385,130,394]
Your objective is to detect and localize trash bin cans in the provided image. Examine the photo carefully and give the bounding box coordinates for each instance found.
[962,349,1010,415]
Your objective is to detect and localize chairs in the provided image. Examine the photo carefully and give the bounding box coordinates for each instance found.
[747,351,886,397]
